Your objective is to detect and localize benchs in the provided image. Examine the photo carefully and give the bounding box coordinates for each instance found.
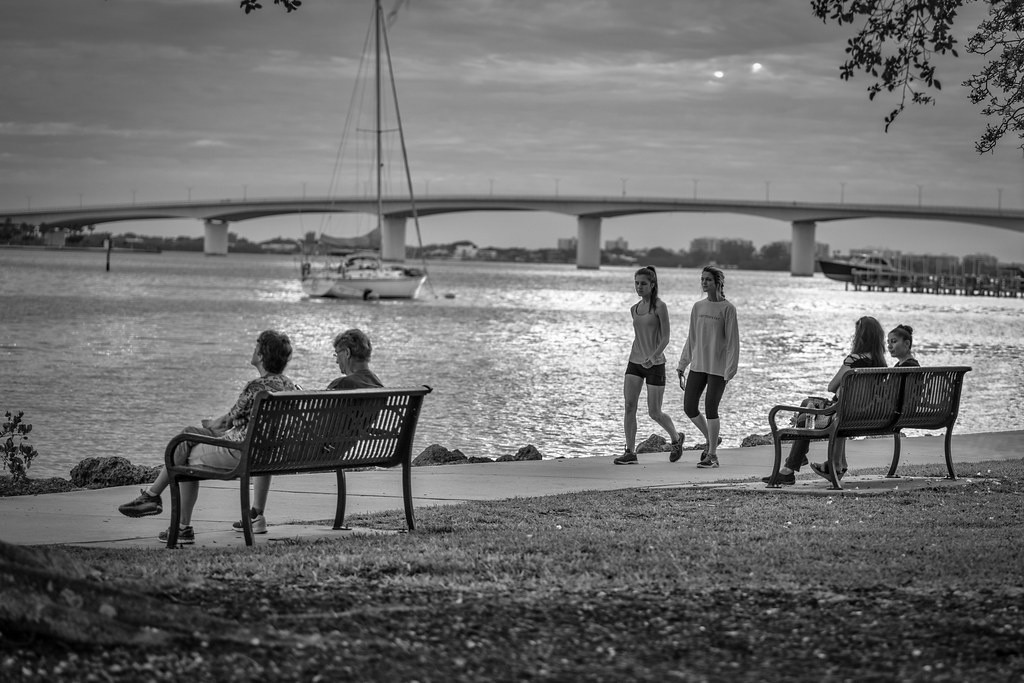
[764,365,974,490]
[164,385,434,550]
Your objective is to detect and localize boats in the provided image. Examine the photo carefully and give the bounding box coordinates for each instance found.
[816,253,930,282]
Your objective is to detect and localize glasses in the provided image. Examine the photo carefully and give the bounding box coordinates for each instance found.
[333,348,345,358]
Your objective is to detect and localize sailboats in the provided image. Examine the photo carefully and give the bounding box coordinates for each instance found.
[296,0,436,299]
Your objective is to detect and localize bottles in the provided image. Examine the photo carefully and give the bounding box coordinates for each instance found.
[806,401,816,429]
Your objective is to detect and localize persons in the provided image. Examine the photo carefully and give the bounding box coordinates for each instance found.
[810,324,922,482]
[230,329,385,533]
[677,266,739,467]
[762,315,888,485]
[119,330,294,544]
[613,266,685,464]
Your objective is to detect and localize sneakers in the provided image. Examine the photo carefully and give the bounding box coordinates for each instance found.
[762,472,795,485]
[614,449,638,464]
[785,454,808,466]
[697,457,720,468]
[158,525,195,544]
[232,512,267,533]
[118,488,163,518]
[701,436,722,460]
[669,433,685,462]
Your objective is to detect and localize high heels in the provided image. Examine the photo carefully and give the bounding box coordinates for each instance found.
[810,461,847,481]
